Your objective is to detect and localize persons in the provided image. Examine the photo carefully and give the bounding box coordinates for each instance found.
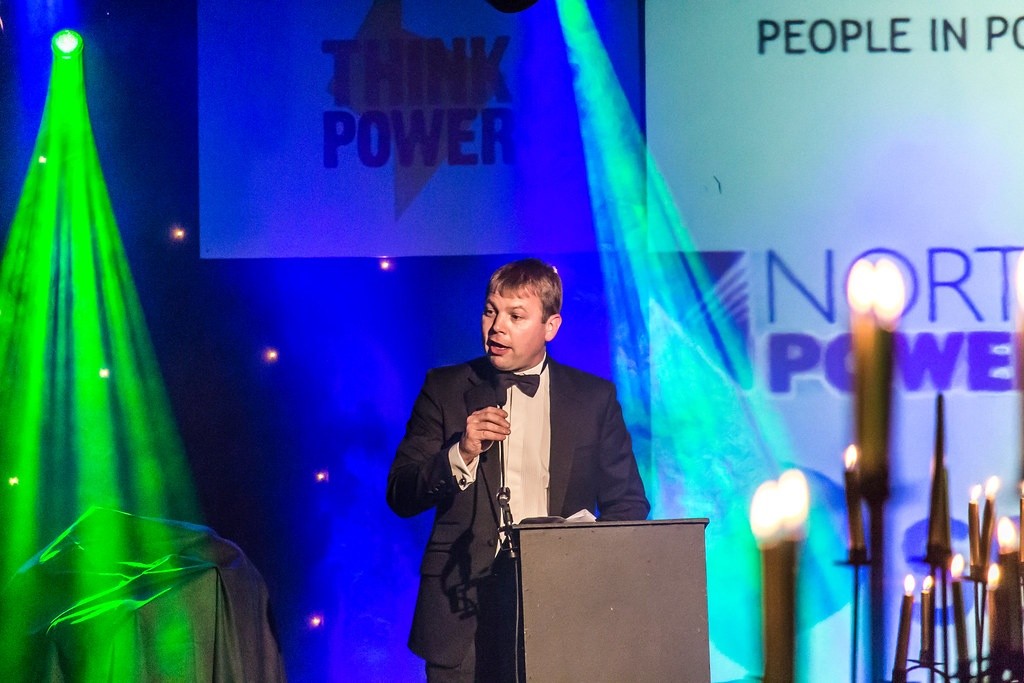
[388,261,649,683]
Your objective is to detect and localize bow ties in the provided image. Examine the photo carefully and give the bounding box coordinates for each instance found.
[493,353,549,398]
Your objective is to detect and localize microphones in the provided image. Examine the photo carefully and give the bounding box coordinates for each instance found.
[496,385,511,504]
